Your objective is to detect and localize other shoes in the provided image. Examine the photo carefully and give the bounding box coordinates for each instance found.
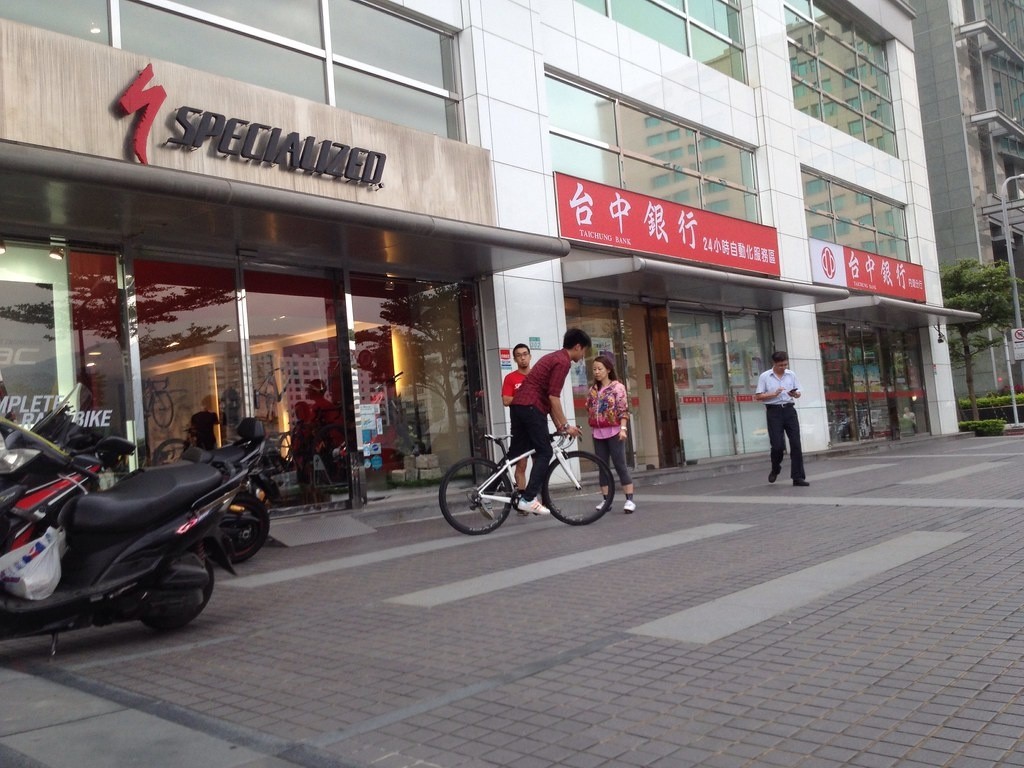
[793,477,809,487]
[768,470,777,483]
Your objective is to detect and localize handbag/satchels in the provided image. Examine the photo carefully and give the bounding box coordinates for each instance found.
[0,524,68,600]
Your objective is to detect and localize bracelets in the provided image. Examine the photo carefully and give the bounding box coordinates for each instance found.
[560,425,562,430]
[621,427,627,431]
[564,423,569,428]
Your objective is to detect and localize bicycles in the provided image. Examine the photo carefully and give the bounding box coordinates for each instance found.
[142,376,174,428]
[439,425,615,535]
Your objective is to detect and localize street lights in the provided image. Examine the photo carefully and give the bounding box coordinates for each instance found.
[1001,174,1024,382]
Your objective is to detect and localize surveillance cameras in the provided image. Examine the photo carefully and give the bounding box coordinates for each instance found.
[938,336,945,343]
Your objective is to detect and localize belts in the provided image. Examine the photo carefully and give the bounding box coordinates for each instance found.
[766,403,793,408]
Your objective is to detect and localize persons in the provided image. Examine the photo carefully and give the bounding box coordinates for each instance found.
[189,406,218,451]
[583,356,637,512]
[478,329,590,519]
[502,343,535,515]
[291,380,345,501]
[756,351,809,486]
[903,408,916,423]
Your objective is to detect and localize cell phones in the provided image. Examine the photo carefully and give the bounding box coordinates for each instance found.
[790,388,798,392]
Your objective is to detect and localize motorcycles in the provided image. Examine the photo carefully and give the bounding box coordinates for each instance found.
[29,383,281,565]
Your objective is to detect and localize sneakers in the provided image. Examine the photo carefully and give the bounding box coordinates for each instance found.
[623,500,636,513]
[478,493,494,520]
[596,500,611,511]
[518,497,550,515]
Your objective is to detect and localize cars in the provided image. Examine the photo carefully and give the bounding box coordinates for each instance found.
[358,447,406,479]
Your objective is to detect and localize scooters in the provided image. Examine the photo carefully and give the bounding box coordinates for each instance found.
[0,417,249,658]
